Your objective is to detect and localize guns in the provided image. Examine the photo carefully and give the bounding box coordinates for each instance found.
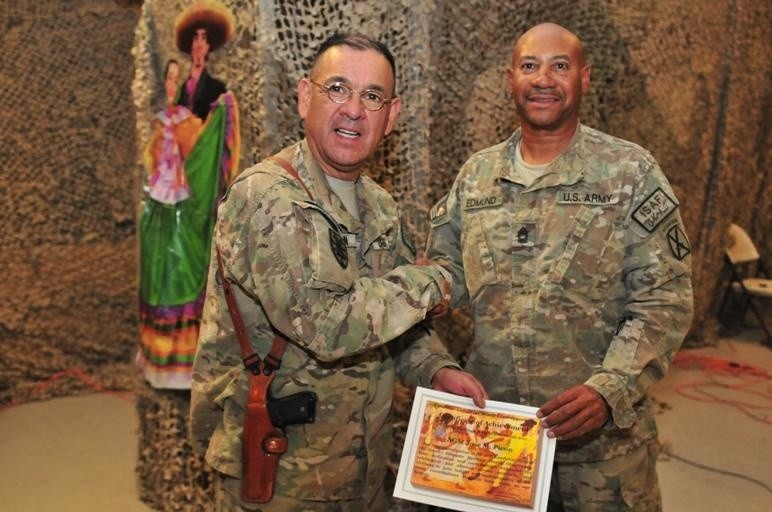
[239,384,317,503]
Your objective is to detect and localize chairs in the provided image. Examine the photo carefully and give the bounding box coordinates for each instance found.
[717,223,772,345]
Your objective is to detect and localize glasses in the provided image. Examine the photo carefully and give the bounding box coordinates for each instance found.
[308,77,393,112]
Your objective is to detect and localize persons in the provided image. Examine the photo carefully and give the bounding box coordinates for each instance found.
[190,35,489,511]
[425,411,537,492]
[416,22,694,512]
